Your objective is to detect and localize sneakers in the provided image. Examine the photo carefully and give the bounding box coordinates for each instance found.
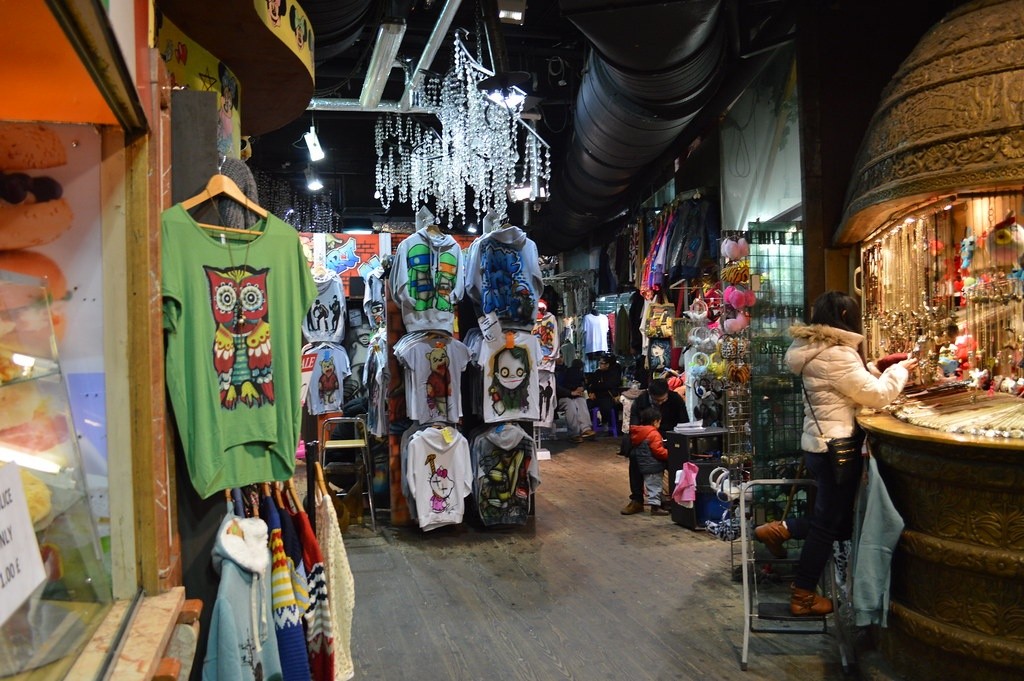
[620,499,643,514]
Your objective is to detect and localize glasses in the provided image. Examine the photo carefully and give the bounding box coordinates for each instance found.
[598,361,607,364]
[652,396,668,402]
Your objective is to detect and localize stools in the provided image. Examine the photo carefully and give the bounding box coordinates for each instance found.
[594,407,617,437]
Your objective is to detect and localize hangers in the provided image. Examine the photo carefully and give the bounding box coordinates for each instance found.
[425,330,450,345]
[498,213,513,229]
[423,216,444,238]
[225,441,329,518]
[318,341,335,349]
[315,258,327,275]
[163,155,298,238]
[670,276,715,289]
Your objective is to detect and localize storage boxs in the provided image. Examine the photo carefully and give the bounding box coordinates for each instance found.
[696,493,731,527]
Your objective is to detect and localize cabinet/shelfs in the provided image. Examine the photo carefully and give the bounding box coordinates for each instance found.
[719,218,804,585]
[666,428,734,531]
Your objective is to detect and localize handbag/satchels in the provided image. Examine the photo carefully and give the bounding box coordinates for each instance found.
[825,435,862,465]
[718,509,741,542]
[649,339,672,369]
[644,295,675,338]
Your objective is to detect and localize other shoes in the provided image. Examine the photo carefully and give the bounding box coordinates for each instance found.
[616,452,629,460]
[569,436,583,442]
[650,508,669,515]
[582,430,596,437]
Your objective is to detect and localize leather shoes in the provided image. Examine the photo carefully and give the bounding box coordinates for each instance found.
[751,521,790,559]
[790,588,841,617]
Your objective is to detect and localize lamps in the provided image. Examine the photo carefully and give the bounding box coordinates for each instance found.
[360,18,405,109]
[305,126,325,161]
[488,84,527,109]
[508,181,547,201]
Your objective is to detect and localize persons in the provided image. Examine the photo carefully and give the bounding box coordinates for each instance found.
[555,350,595,443]
[620,377,690,515]
[566,359,585,385]
[630,408,670,516]
[753,292,918,615]
[588,355,622,432]
[943,319,965,346]
